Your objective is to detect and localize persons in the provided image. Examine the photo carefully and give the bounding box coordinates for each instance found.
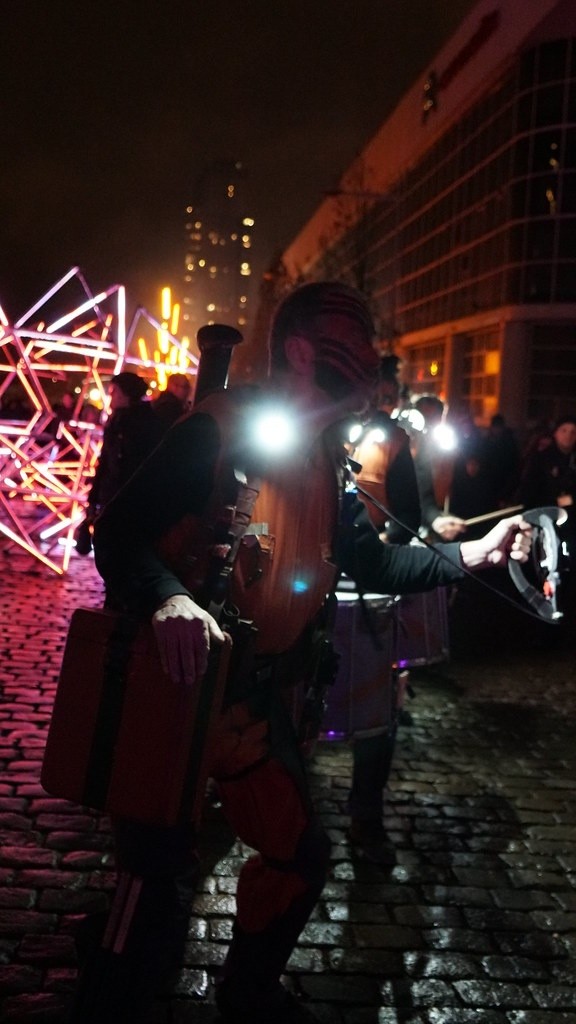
[320,376,464,864]
[84,370,156,616]
[409,393,574,667]
[152,373,190,423]
[93,280,538,1022]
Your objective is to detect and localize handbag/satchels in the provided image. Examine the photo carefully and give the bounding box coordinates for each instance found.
[39,606,232,834]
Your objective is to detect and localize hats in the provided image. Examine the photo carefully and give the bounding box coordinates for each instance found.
[112,372,148,398]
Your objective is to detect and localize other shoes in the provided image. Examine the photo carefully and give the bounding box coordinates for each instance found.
[215,937,321,1024]
[346,820,398,873]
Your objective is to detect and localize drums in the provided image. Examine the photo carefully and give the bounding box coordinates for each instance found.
[394,587,452,668]
[317,590,405,748]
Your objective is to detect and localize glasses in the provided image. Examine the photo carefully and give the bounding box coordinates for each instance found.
[178,384,189,390]
